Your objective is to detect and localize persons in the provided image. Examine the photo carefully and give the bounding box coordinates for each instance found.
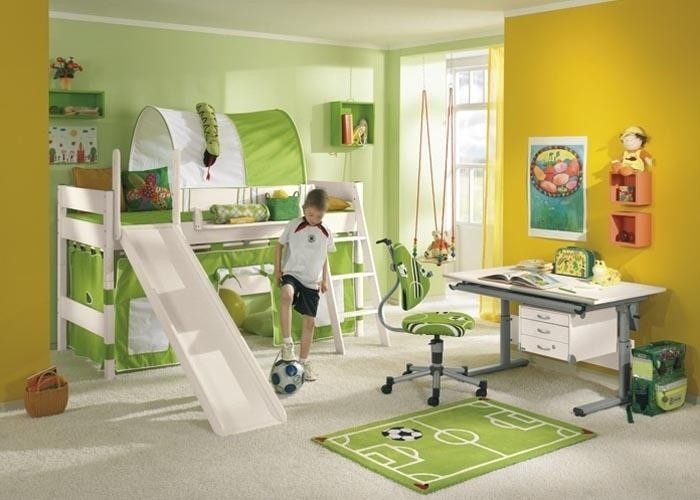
[275,189,338,382]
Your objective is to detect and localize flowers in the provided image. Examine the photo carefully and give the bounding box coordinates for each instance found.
[50,55,84,80]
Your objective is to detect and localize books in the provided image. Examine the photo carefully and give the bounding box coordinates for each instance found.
[516,262,553,271]
[477,272,563,290]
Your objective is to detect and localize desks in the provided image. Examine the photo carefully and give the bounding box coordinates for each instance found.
[439,261,666,416]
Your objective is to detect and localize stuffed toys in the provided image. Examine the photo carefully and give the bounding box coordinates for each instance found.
[612,123,652,178]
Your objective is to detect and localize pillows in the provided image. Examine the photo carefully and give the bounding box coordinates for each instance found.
[123,166,175,212]
[71,166,126,216]
[308,194,350,212]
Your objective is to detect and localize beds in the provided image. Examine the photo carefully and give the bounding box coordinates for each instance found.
[57,109,391,440]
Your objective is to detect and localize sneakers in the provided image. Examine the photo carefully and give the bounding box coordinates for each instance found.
[299,361,316,381]
[359,119,368,144]
[281,342,297,362]
[353,124,365,145]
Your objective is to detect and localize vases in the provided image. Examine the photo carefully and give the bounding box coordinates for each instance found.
[59,77,74,90]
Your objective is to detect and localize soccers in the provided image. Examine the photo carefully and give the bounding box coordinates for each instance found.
[271,360,304,394]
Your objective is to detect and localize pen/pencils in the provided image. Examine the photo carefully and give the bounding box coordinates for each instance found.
[558,287,576,294]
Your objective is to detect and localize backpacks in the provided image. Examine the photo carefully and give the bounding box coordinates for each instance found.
[626,340,688,423]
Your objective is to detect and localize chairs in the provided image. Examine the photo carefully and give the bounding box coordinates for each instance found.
[371,232,491,406]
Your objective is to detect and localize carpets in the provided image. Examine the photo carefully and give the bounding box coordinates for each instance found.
[310,398,597,494]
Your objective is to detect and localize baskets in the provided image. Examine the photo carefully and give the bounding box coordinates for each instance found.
[265,191,301,220]
[24,371,68,418]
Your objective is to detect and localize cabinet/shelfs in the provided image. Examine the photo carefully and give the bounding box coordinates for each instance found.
[329,101,375,147]
[49,89,105,119]
[517,305,621,363]
[607,169,649,250]
